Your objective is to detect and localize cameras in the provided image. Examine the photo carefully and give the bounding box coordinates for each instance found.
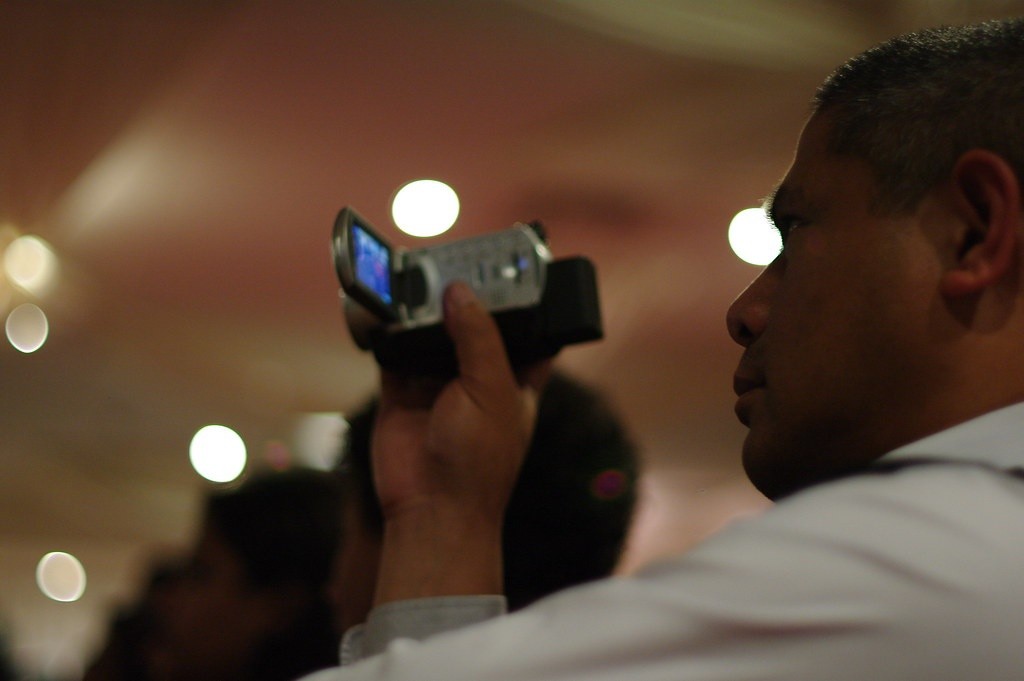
[331,206,605,409]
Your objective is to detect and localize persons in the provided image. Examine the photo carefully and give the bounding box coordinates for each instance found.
[307,14,1024,680]
[83,370,642,681]
